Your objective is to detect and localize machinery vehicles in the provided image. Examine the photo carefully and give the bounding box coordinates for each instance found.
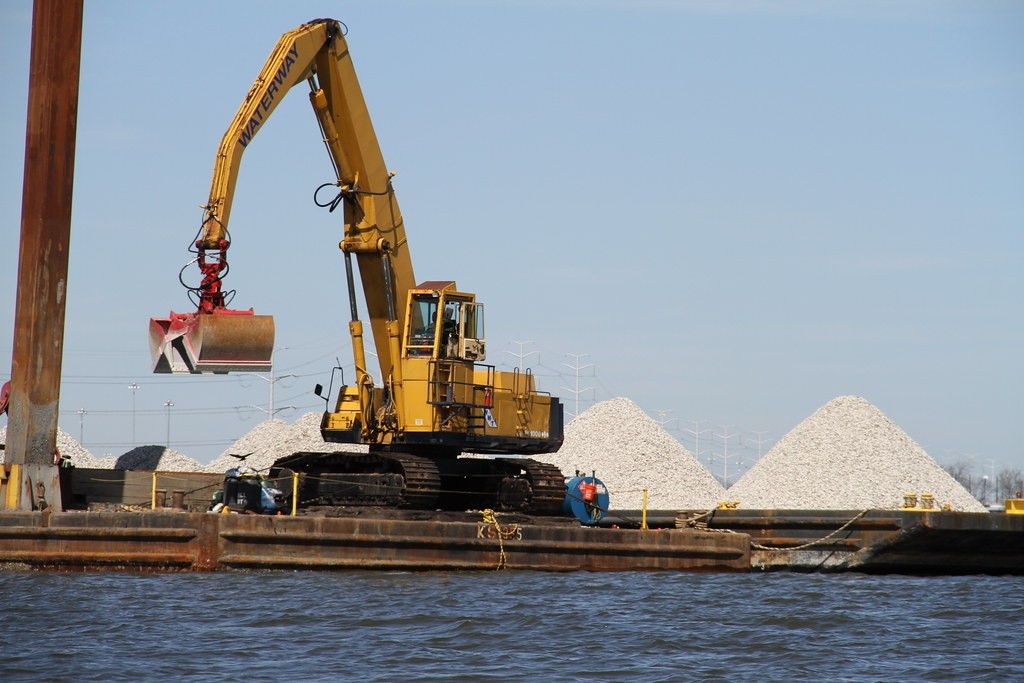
[150,18,569,518]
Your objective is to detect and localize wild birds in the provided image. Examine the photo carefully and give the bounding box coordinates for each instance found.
[229,449,260,461]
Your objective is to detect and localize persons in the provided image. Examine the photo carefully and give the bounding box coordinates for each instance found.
[422,309,439,336]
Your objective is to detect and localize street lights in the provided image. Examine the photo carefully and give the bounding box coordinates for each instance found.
[163,399,175,449]
[75,408,87,448]
[127,382,141,449]
